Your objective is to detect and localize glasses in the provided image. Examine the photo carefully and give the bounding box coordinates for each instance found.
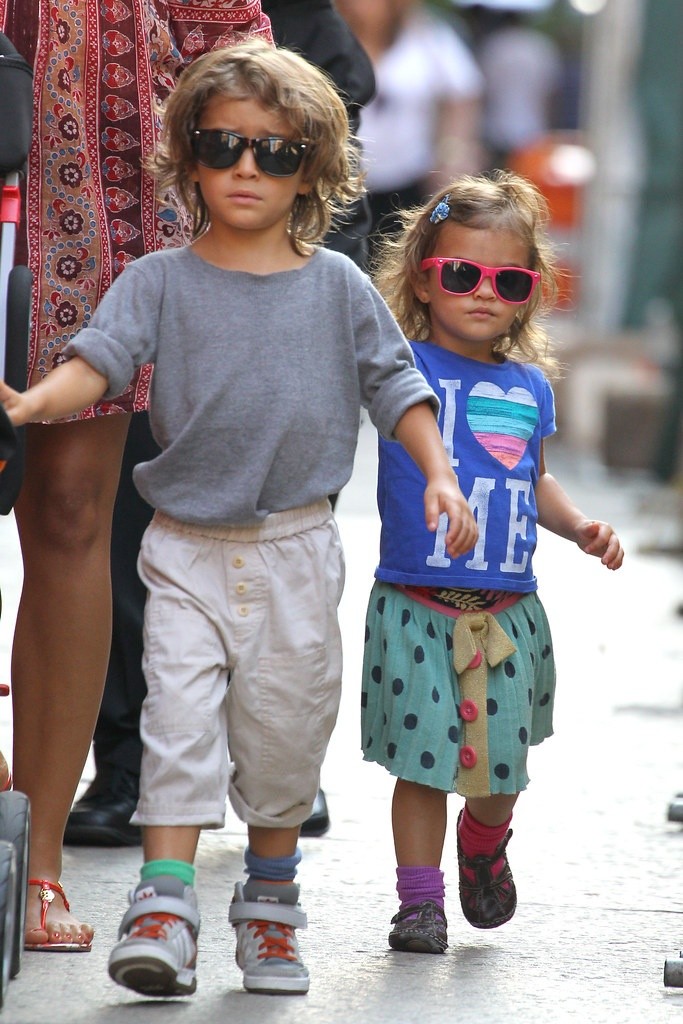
[190,128,309,177]
[420,256,542,306]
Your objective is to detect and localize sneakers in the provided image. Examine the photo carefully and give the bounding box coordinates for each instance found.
[103,874,202,997]
[223,879,312,993]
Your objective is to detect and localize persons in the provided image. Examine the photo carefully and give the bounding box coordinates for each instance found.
[466,6,565,172]
[0,42,482,997]
[360,176,625,954]
[330,0,484,279]
[0,0,276,953]
[64,0,379,847]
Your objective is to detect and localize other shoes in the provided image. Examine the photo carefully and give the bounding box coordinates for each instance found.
[64,773,142,848]
[298,788,331,837]
[388,900,449,954]
[456,809,519,931]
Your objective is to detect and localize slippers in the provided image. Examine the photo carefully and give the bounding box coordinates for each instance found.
[23,878,95,952]
